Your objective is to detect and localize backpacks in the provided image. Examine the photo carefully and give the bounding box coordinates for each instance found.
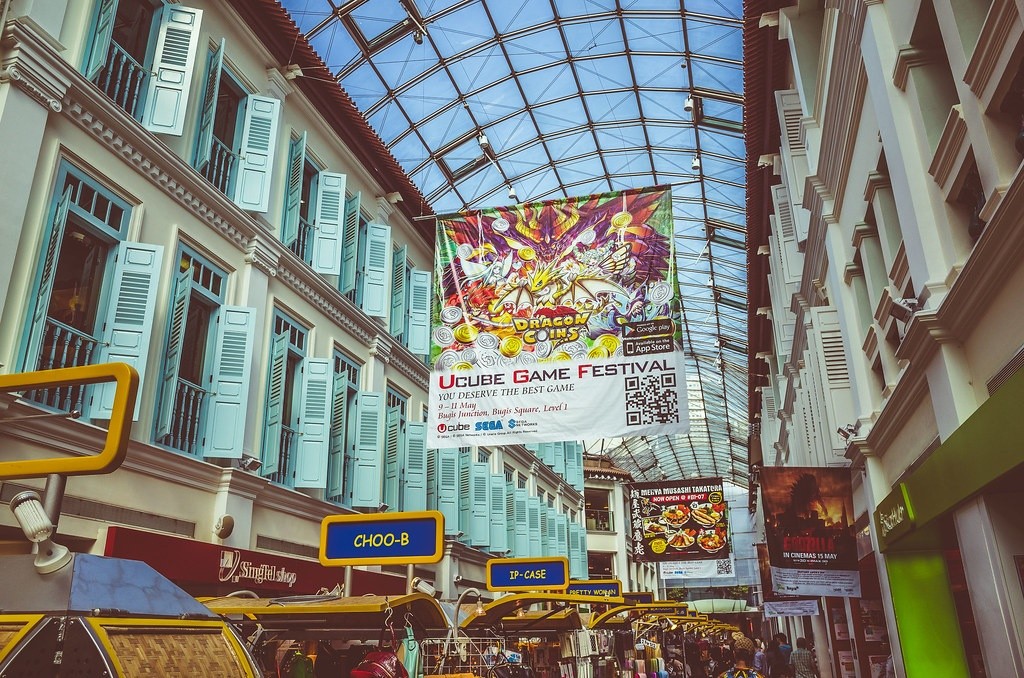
[350,624,423,678]
[486,651,536,678]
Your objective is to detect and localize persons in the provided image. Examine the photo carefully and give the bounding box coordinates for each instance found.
[711,646,731,678]
[765,640,786,678]
[749,638,768,678]
[755,639,764,653]
[686,643,707,678]
[789,637,821,678]
[717,637,766,678]
[776,633,795,678]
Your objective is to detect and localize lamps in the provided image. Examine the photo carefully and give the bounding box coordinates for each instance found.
[456,531,463,538]
[479,135,489,148]
[9,491,72,576]
[707,276,714,288]
[713,337,720,348]
[378,503,389,513]
[500,549,511,557]
[888,297,920,322]
[837,424,855,439]
[454,587,487,636]
[241,458,263,471]
[684,94,694,111]
[410,577,437,598]
[508,187,517,198]
[494,593,527,619]
[691,154,700,170]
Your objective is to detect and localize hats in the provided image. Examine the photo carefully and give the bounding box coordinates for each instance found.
[734,637,755,655]
